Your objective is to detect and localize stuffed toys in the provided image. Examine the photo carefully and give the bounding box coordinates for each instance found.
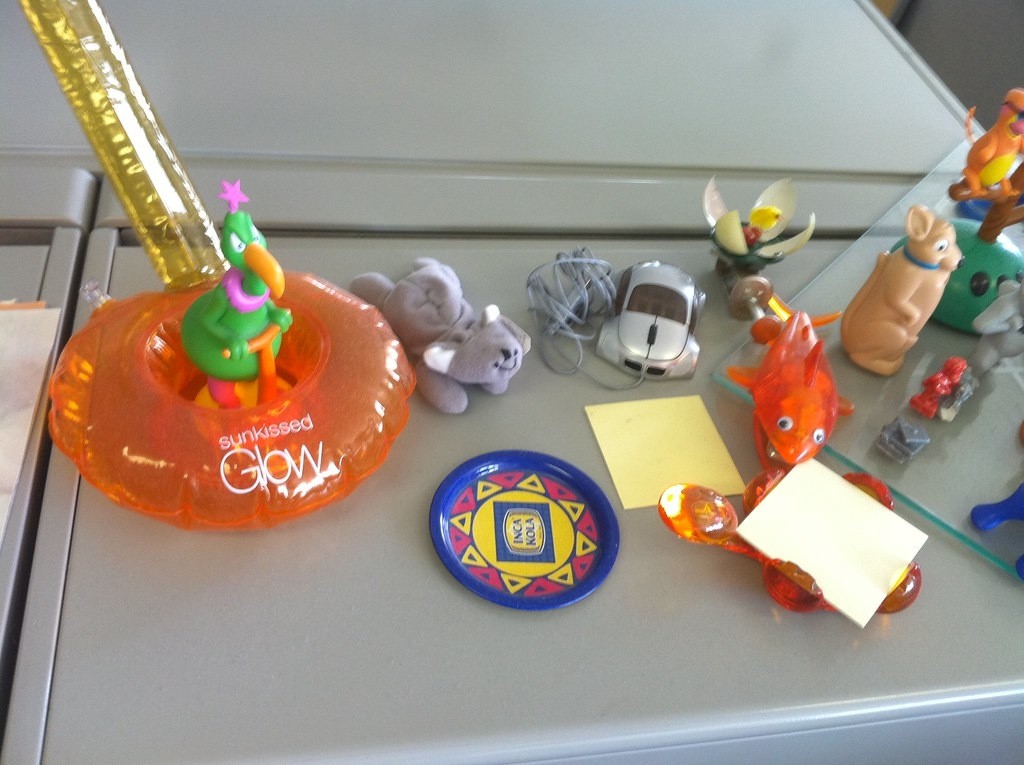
[350,257,527,414]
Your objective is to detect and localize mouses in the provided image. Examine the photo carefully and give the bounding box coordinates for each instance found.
[595,261,706,380]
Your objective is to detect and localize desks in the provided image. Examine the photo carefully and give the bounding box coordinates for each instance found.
[0,0,1024,765]
